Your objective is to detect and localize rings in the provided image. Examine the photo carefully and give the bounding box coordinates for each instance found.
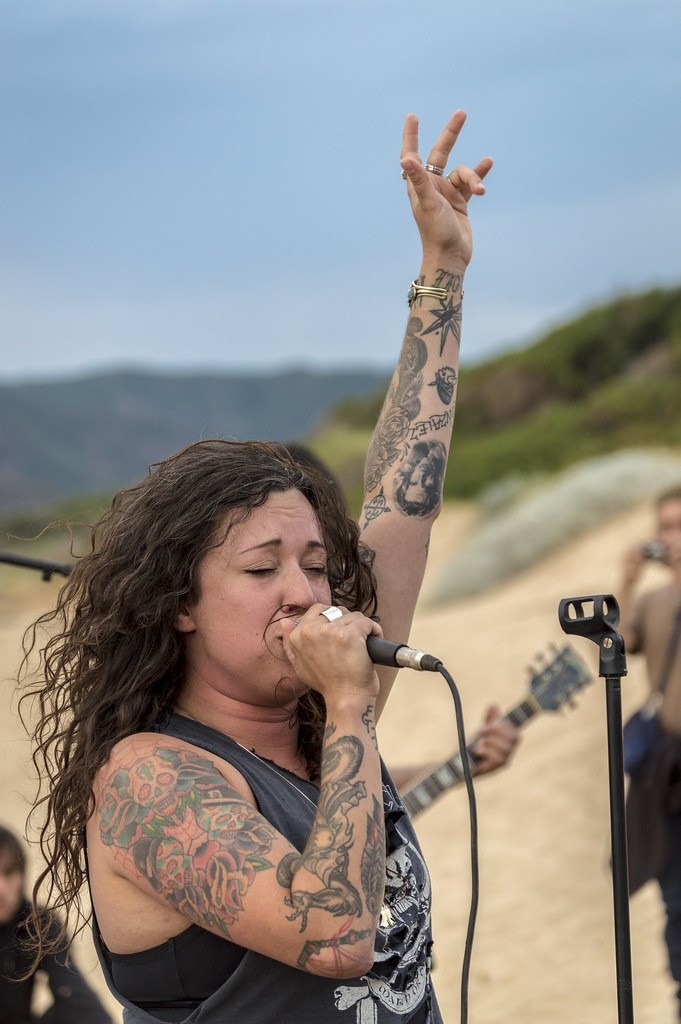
[445,174,460,190]
[424,164,444,176]
[319,606,343,622]
[401,168,407,180]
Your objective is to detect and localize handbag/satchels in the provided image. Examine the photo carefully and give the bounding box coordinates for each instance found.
[623,710,659,771]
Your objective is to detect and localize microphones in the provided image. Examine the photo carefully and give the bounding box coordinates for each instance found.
[367,634,443,673]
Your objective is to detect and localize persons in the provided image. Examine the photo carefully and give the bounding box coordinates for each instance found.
[386,703,518,796]
[0,825,113,1024]
[5,108,522,1024]
[613,483,681,1024]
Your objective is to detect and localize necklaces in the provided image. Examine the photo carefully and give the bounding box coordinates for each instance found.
[175,704,395,928]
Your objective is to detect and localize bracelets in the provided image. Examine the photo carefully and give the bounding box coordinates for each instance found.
[407,278,465,307]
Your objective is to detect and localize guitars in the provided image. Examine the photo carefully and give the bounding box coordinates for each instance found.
[398,638,596,826]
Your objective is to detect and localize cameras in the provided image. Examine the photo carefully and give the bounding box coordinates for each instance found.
[643,540,669,562]
